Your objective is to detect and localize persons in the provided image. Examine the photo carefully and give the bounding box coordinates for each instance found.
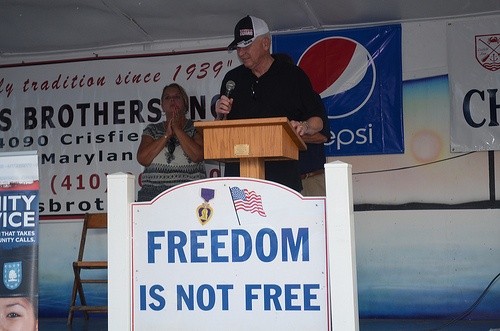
[213,15,332,196]
[136,83,208,202]
[0,295,38,331]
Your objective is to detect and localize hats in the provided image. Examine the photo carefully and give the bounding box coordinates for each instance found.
[227,15,269,49]
[0,244,39,298]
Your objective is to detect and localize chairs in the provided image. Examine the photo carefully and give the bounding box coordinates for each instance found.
[67,212,108,324]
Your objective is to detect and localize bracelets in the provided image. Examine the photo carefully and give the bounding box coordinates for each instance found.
[163,135,170,140]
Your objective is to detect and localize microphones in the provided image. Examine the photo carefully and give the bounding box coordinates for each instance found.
[220,80,235,120]
